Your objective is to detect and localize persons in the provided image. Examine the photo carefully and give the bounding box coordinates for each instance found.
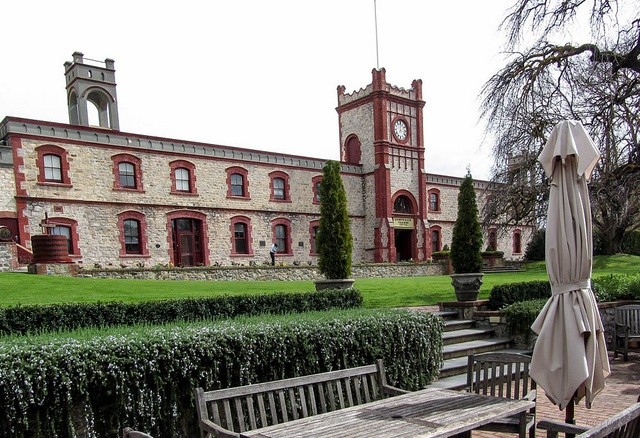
[270,243,278,266]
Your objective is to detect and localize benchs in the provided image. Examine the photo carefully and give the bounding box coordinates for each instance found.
[537,397,640,438]
[193,356,412,437]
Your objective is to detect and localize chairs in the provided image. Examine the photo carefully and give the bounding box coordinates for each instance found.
[458,351,537,437]
[614,304,640,361]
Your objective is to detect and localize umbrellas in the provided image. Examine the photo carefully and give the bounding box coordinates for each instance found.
[528,119,612,438]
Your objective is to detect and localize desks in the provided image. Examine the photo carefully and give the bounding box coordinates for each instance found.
[240,387,536,438]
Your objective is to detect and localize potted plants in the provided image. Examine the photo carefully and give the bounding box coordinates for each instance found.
[449,169,485,301]
[313,160,355,290]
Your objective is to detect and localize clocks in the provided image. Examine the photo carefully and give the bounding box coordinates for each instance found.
[392,116,410,143]
